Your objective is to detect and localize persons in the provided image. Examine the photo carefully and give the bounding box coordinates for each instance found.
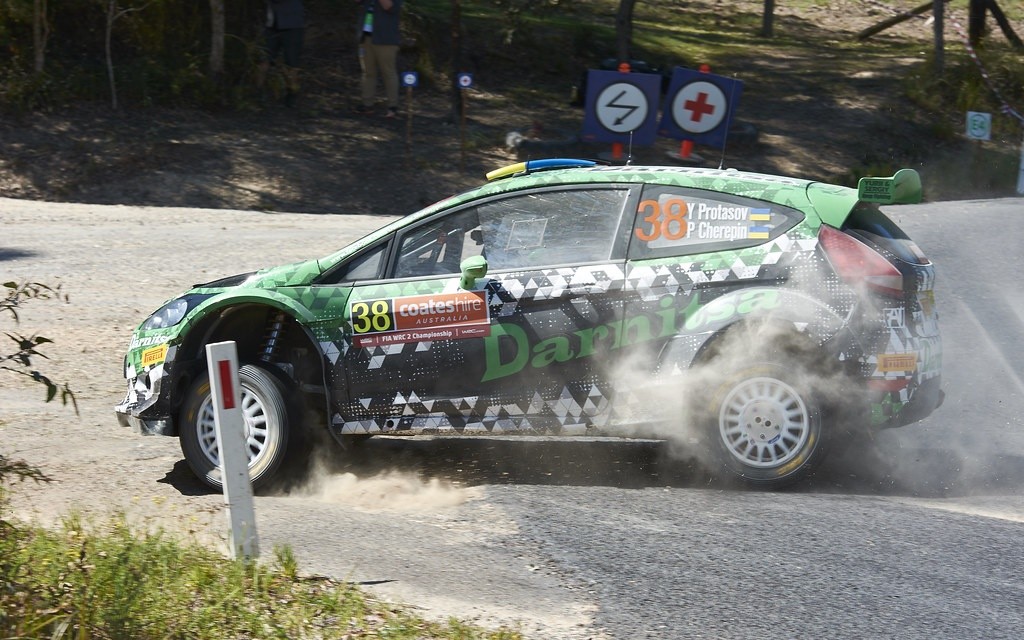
[356,0,401,120]
[273,1,307,73]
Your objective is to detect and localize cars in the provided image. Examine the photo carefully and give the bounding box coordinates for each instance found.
[113,166,947,498]
